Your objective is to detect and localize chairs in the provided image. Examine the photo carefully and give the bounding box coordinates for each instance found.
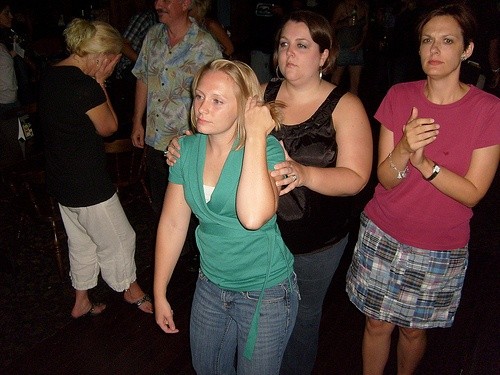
[104,139,153,206]
[16,171,67,288]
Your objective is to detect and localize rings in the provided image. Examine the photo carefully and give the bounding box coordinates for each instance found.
[289,175,297,182]
[164,151,169,156]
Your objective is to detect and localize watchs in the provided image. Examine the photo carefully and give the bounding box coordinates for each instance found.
[425,161,440,182]
[425,161,440,183]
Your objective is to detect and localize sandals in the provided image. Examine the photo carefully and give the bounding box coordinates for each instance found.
[71,302,110,325]
[119,294,154,316]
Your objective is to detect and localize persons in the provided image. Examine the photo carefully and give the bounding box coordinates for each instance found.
[166,12,373,375]
[28,17,154,321]
[0,0,25,158]
[346,4,500,375]
[153,59,301,375]
[115,0,500,187]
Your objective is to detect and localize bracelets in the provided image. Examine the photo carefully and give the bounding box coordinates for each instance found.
[388,152,409,179]
[388,153,408,179]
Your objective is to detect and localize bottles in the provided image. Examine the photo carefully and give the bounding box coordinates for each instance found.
[352,8,357,27]
[10,29,23,40]
